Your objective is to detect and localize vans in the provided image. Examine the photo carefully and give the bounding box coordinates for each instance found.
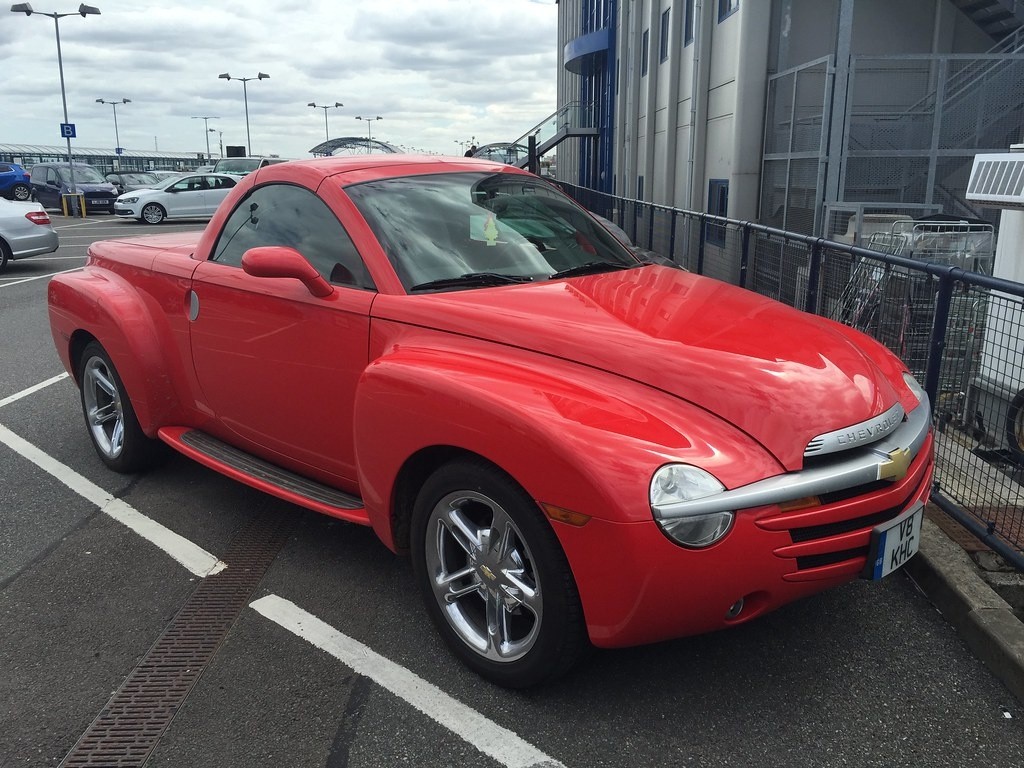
[209,155,290,188]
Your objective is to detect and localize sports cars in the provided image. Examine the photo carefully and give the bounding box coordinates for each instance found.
[48,153,938,694]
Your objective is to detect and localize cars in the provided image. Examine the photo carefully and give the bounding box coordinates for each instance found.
[111,165,226,194]
[0,197,60,271]
[113,172,243,225]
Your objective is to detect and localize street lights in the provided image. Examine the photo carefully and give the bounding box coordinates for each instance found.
[356,116,384,153]
[307,102,344,156]
[218,72,270,157]
[95,98,133,173]
[10,2,102,215]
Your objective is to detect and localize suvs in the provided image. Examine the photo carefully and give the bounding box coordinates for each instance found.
[29,162,120,217]
[0,160,33,201]
